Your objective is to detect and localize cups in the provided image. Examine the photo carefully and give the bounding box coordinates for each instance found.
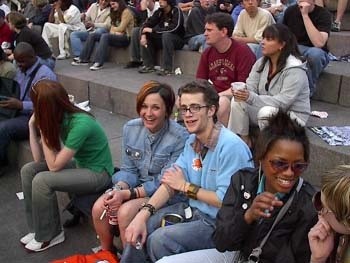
[231,82,246,102]
[108,204,120,225]
[162,215,183,227]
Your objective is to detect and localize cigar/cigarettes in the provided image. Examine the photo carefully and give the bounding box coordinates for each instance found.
[100,206,108,220]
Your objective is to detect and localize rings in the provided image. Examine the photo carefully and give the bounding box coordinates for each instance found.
[310,228,314,232]
[109,192,112,196]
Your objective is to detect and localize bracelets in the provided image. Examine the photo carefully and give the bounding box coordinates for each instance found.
[139,203,156,215]
[112,184,122,190]
[183,181,200,199]
[130,187,140,200]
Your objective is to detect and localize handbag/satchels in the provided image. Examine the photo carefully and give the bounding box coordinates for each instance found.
[0,76,20,120]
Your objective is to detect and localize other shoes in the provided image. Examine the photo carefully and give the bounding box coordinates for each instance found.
[123,61,140,69]
[56,53,69,60]
[71,60,88,66]
[73,56,79,62]
[138,64,154,73]
[331,20,342,32]
[247,134,257,157]
[89,62,104,70]
[155,68,172,76]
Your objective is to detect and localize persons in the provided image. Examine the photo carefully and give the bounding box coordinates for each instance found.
[153,105,319,263]
[0,0,350,177]
[91,79,189,252]
[308,164,350,263]
[119,78,256,263]
[19,80,114,252]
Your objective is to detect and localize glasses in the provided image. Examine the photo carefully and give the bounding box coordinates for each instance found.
[32,77,49,94]
[265,158,308,173]
[312,191,333,215]
[177,105,208,115]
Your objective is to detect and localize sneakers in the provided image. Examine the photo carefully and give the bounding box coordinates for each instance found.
[19,228,65,252]
